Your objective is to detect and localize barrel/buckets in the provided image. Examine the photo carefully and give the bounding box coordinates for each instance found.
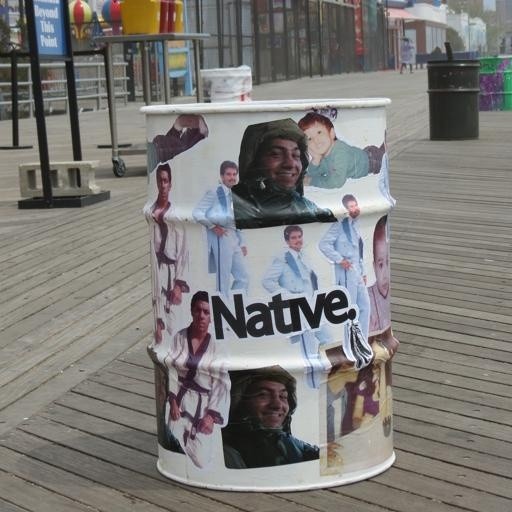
[472,56,507,113]
[497,55,512,111]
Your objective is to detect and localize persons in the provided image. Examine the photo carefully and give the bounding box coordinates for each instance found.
[145,108,401,473]
[398,37,416,75]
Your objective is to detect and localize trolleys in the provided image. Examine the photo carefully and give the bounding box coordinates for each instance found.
[94,31,211,181]
